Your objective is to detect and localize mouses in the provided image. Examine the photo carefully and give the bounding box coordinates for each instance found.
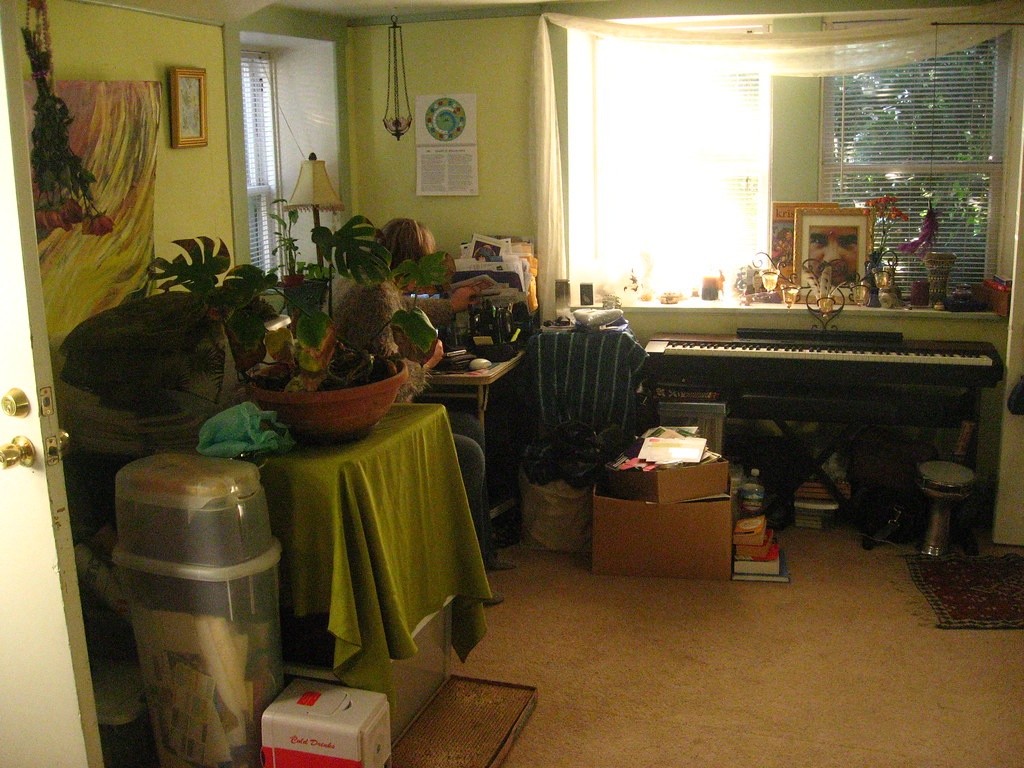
[469,359,492,371]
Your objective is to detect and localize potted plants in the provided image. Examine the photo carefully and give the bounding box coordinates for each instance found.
[269,198,306,285]
[144,215,409,449]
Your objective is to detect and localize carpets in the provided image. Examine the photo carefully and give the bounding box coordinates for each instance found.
[897,552,1024,630]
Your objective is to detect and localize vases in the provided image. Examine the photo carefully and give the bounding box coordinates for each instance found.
[866,293,879,308]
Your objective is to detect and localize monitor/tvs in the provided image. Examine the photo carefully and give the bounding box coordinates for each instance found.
[406,289,448,343]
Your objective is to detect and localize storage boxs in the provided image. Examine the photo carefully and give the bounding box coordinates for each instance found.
[590,485,733,582]
[259,678,391,768]
[735,529,774,557]
[794,499,839,530]
[733,515,767,545]
[605,457,729,504]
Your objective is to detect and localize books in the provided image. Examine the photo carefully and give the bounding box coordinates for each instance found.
[793,470,843,532]
[730,515,791,583]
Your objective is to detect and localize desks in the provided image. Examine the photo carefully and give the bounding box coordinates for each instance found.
[205,401,492,743]
[421,350,526,448]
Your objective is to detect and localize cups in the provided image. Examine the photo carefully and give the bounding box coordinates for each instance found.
[910,280,930,306]
[701,277,718,301]
[580,281,594,305]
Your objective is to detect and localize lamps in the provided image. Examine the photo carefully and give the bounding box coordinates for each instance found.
[282,152,345,263]
[382,14,413,142]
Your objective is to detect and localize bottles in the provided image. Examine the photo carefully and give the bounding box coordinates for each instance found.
[738,466,763,519]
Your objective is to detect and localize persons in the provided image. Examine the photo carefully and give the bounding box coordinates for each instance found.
[810,225,859,286]
[372,216,519,571]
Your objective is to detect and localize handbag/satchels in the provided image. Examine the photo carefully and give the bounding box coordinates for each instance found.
[862,503,921,551]
[196,402,297,458]
[525,419,605,491]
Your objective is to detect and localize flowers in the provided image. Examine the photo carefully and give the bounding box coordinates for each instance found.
[863,194,909,263]
[21,25,116,238]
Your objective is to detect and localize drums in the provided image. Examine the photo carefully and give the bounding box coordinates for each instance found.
[917,460,978,562]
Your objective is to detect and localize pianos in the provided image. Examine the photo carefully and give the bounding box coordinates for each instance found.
[642,330,1005,534]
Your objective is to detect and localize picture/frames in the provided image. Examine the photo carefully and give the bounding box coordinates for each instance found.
[794,207,875,305]
[168,66,209,148]
